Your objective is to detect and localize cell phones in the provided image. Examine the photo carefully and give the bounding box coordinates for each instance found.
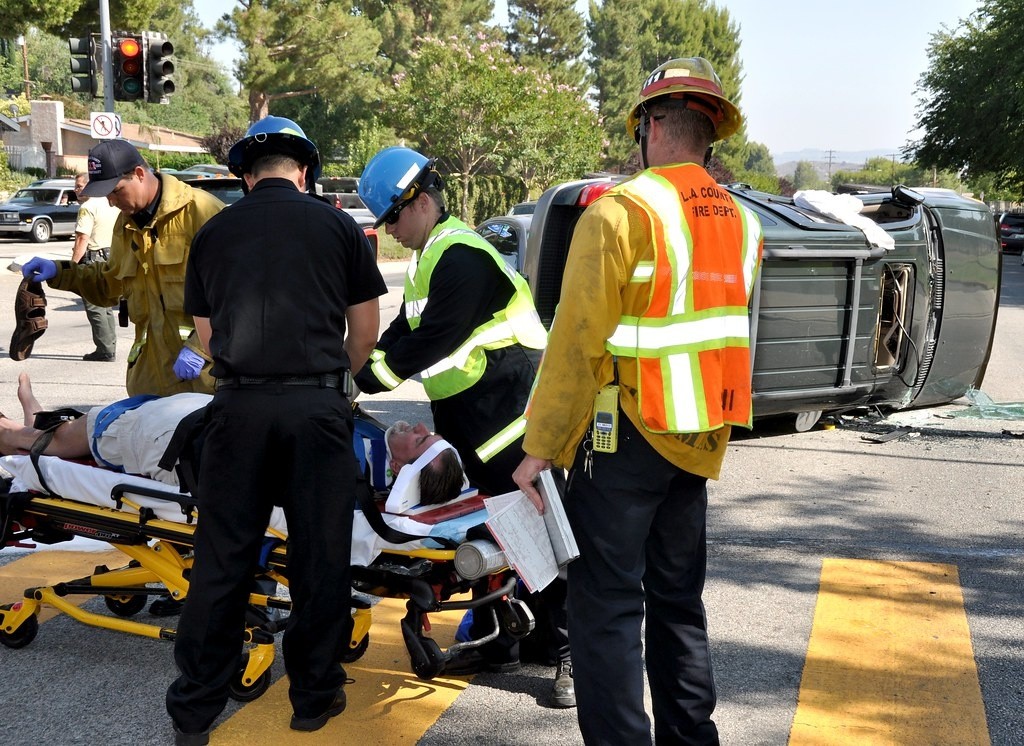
[593,385,621,452]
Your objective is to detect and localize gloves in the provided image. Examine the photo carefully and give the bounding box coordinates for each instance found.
[22,257,56,282]
[346,379,361,405]
[172,346,205,381]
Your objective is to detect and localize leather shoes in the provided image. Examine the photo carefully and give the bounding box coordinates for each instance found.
[550,660,576,706]
[290,687,347,732]
[439,641,520,675]
[83,351,115,362]
[175,730,209,746]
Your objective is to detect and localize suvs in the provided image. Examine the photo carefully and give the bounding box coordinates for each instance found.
[0,179,81,243]
[993,211,1024,253]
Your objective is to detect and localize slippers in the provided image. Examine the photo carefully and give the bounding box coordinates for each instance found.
[9,272,48,361]
[0,412,8,456]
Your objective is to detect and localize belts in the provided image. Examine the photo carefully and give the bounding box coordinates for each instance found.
[83,248,109,257]
[217,374,340,388]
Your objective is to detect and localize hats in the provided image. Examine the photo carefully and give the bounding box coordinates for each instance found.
[79,139,145,197]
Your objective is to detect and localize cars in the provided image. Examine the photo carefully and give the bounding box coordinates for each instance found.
[508,201,537,214]
[475,215,534,276]
[526,169,1003,432]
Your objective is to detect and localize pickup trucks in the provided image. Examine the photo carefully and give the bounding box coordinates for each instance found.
[314,177,366,210]
[186,175,378,268]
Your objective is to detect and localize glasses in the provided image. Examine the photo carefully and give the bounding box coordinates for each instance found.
[385,174,436,225]
[634,114,666,144]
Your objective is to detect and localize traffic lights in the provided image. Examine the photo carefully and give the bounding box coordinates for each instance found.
[68,35,96,96]
[111,35,144,102]
[142,30,176,105]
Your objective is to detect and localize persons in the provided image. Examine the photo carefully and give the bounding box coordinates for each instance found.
[165,115,389,746]
[71,171,122,362]
[0,371,466,508]
[23,139,278,622]
[347,147,577,708]
[514,56,762,746]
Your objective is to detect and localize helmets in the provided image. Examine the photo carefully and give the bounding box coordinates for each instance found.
[626,57,742,143]
[228,116,321,192]
[358,147,436,230]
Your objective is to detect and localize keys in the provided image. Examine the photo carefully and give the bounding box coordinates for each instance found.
[584,450,594,479]
